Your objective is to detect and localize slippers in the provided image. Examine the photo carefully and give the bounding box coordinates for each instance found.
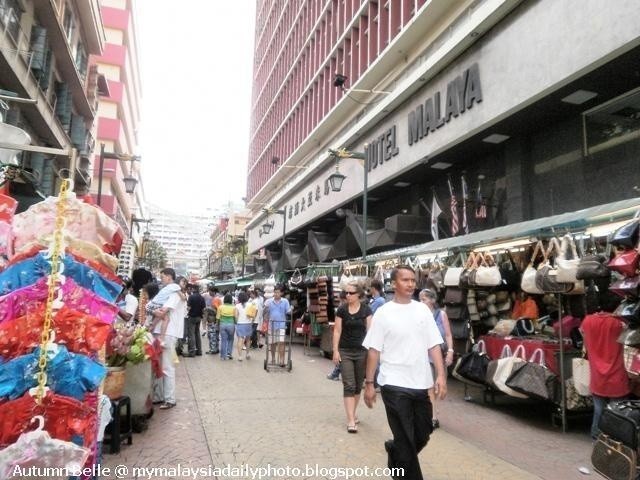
[346,414,361,433]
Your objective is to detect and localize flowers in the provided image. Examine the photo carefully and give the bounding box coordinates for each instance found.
[106,322,145,368]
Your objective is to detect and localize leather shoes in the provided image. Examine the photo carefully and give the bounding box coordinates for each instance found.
[383,437,395,478]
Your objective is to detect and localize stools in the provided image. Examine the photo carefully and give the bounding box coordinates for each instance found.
[103,395,133,453]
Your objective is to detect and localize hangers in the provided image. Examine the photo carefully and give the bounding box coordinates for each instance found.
[21,177,77,442]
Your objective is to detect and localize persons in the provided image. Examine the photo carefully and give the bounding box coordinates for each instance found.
[578,290,634,441]
[115,264,298,409]
[325,264,454,480]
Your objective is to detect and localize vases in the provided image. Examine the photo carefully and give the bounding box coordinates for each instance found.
[101,366,127,400]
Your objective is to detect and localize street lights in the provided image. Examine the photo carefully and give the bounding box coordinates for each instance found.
[95,143,141,216]
[129,212,154,243]
[227,234,246,281]
[260,204,288,271]
[326,146,372,262]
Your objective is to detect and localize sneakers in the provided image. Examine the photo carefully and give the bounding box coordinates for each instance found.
[152,398,165,404]
[159,401,177,410]
[431,417,440,429]
[327,373,339,381]
[182,343,264,361]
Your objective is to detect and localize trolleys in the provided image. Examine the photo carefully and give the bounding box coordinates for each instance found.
[105,359,155,436]
[261,306,298,373]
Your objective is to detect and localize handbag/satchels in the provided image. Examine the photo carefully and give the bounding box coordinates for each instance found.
[609,295,640,326]
[619,342,640,383]
[492,344,530,399]
[597,399,640,451]
[605,272,639,300]
[565,376,594,412]
[504,348,559,400]
[591,432,639,480]
[485,344,512,394]
[606,247,640,278]
[451,356,486,388]
[570,356,593,397]
[612,321,640,346]
[246,231,608,344]
[455,340,493,384]
[608,209,640,250]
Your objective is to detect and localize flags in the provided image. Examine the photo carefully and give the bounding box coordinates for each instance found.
[449,188,459,237]
[475,186,486,219]
[430,195,442,240]
[462,182,471,235]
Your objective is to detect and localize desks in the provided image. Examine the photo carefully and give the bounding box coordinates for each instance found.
[478,335,574,407]
